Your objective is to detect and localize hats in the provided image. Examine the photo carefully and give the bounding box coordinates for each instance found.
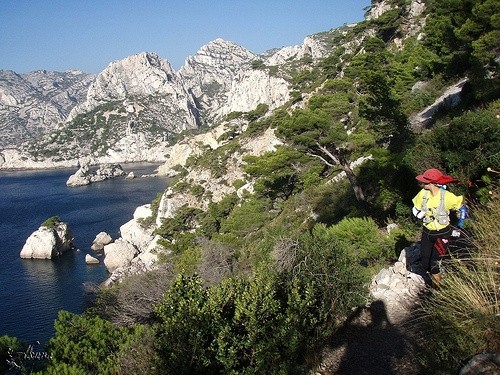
[415,169,442,183]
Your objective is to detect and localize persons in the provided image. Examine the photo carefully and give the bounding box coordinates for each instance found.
[411,168,469,282]
[64,234,76,250]
